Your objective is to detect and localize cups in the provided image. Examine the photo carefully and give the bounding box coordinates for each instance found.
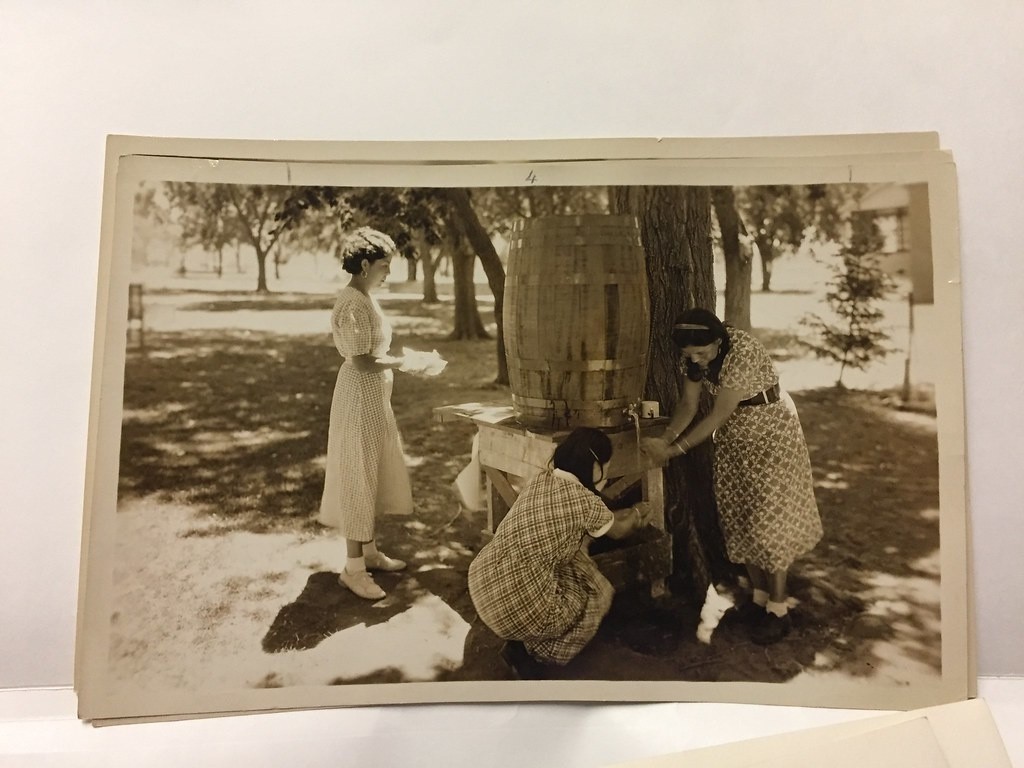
[642,401,659,419]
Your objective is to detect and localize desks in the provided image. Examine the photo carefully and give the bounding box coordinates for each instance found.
[432,397,674,601]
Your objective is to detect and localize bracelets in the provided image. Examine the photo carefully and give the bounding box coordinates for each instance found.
[632,505,640,518]
[660,426,691,455]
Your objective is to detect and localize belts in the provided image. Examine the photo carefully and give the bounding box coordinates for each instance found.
[738,383,781,406]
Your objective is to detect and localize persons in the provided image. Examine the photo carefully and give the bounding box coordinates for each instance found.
[467,427,653,680]
[644,308,824,645]
[319,226,413,601]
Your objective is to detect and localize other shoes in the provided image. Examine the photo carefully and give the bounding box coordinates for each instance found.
[498,640,547,680]
[724,600,766,620]
[750,611,792,643]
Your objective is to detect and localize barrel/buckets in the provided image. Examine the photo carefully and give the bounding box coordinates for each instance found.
[502,215,652,426]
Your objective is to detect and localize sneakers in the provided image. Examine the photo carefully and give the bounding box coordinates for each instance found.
[365,552,407,571]
[339,567,387,600]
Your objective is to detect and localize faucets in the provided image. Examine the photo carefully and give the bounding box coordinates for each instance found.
[623,403,639,425]
